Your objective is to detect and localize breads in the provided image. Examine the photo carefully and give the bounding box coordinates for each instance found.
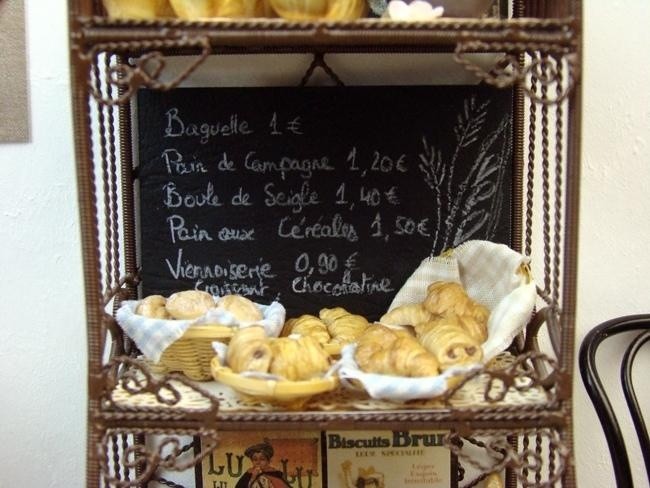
[135,281,490,377]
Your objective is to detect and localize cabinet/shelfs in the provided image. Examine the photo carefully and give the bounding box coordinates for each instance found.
[64,1,581,488]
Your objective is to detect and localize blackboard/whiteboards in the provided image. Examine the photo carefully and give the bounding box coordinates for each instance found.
[135,84,514,323]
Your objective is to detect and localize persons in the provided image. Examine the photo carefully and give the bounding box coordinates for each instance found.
[339,458,387,487]
[232,441,295,488]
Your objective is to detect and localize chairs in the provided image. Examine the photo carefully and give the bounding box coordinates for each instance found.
[578,311,647,488]
